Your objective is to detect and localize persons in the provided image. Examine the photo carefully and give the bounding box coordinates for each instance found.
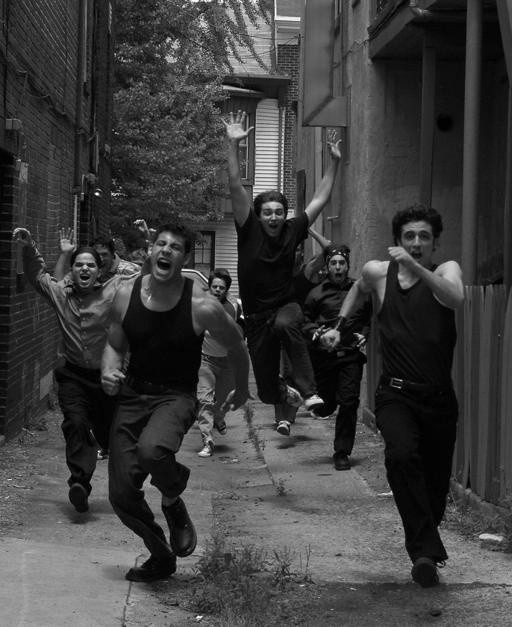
[57,219,152,440]
[125,228,157,262]
[274,227,332,434]
[198,268,243,457]
[219,110,343,411]
[12,227,151,512]
[102,221,249,581]
[305,244,371,471]
[318,203,465,588]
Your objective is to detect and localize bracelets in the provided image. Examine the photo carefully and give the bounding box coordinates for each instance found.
[334,317,346,331]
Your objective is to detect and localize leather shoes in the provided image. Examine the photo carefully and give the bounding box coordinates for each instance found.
[161,496,197,557]
[126,556,176,582]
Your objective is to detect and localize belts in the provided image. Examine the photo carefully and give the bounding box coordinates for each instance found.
[326,350,350,357]
[380,374,430,392]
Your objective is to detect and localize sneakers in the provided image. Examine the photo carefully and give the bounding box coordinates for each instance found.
[197,375,351,470]
[69,483,89,513]
[411,556,439,588]
